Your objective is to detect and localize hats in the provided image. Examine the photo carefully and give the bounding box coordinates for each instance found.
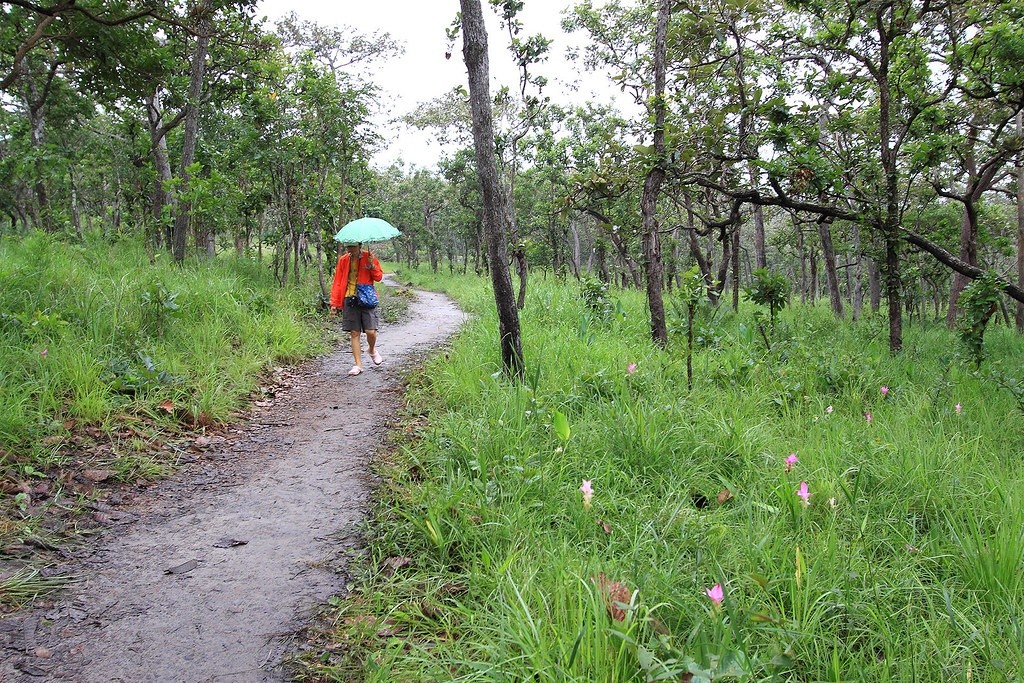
[347,242,359,246]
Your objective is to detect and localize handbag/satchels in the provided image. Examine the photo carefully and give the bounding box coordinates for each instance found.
[355,283,379,309]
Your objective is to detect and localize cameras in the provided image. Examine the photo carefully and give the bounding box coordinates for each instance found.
[347,296,357,307]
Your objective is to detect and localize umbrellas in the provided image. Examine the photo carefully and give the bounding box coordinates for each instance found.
[333,216,402,269]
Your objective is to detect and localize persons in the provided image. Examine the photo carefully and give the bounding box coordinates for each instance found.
[329,241,383,376]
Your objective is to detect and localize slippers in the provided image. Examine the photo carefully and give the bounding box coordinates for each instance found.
[367,349,382,365]
[348,366,363,376]
